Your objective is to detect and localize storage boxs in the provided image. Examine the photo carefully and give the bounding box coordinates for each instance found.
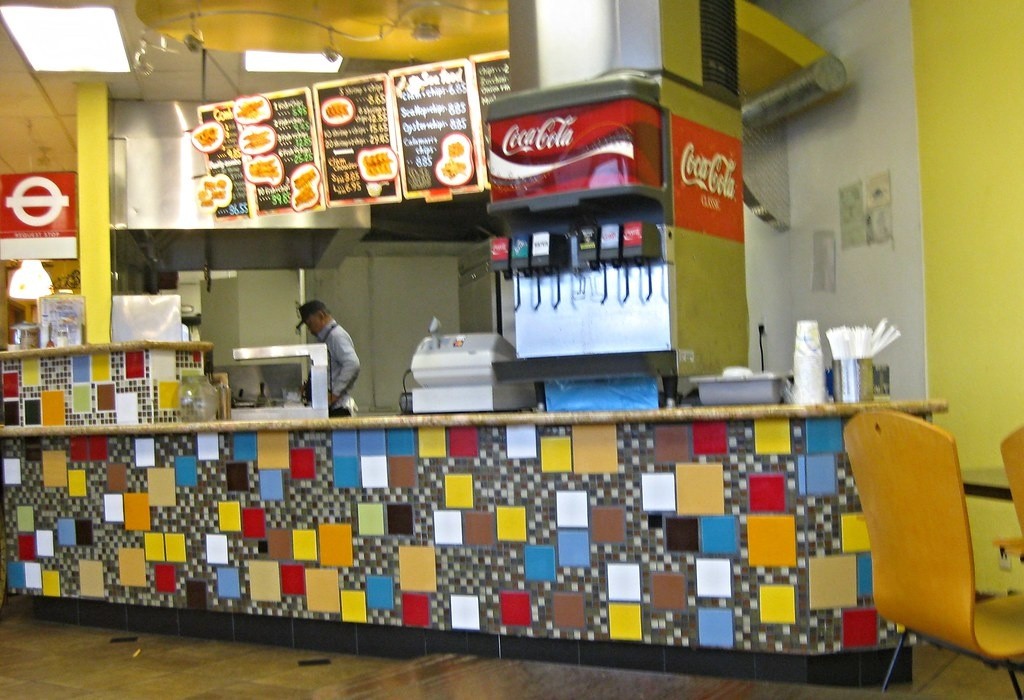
[691,376,779,404]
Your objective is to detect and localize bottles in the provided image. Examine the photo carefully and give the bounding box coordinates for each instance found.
[173,375,219,423]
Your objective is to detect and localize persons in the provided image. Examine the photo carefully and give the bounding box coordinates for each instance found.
[297,300,360,417]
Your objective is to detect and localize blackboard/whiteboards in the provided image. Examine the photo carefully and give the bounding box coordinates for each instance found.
[312,73,403,209]
[197,100,252,223]
[387,58,484,200]
[236,86,327,219]
[468,50,512,191]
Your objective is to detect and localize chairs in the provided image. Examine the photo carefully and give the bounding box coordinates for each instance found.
[842,408,1024,700]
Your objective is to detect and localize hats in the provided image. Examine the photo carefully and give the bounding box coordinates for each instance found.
[297,301,326,329]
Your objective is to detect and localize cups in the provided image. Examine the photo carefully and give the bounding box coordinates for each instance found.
[10,321,39,351]
[832,358,874,405]
[215,386,232,421]
[793,320,828,404]
